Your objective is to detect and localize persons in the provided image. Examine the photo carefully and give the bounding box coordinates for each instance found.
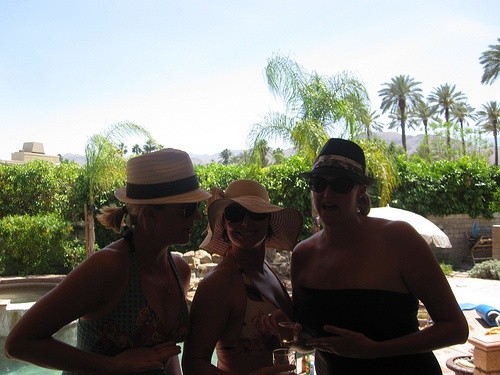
[5,149,213,375]
[182,178,315,375]
[259,138,469,375]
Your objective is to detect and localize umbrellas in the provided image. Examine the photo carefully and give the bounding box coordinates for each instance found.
[367,203,453,249]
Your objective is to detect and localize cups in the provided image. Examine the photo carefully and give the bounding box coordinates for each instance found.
[271,348,297,374]
[279,321,296,347]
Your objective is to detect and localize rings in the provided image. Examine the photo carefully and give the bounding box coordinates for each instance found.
[268,312,272,318]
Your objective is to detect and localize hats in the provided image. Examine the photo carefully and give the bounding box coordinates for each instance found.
[198,181,304,257]
[115,148,213,205]
[297,137,375,189]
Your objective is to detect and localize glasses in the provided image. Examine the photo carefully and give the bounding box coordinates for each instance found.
[306,175,364,193]
[223,206,270,223]
[156,202,200,218]
